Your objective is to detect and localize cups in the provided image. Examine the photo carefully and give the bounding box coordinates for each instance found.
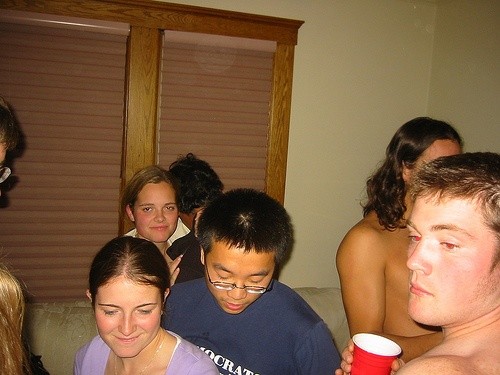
[349,333,402,375]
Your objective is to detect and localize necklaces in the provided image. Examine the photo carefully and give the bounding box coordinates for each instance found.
[112,345,161,375]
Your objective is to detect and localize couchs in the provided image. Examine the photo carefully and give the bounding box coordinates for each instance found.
[24,287,350,375]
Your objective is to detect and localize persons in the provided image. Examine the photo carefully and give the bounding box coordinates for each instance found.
[120,153,225,285]
[0,262,50,375]
[73,236,221,375]
[161,188,341,375]
[0,97,22,196]
[335,150,500,375]
[336,116,464,364]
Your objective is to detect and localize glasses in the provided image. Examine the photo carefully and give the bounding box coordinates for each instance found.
[203,251,275,295]
[0,167,12,183]
